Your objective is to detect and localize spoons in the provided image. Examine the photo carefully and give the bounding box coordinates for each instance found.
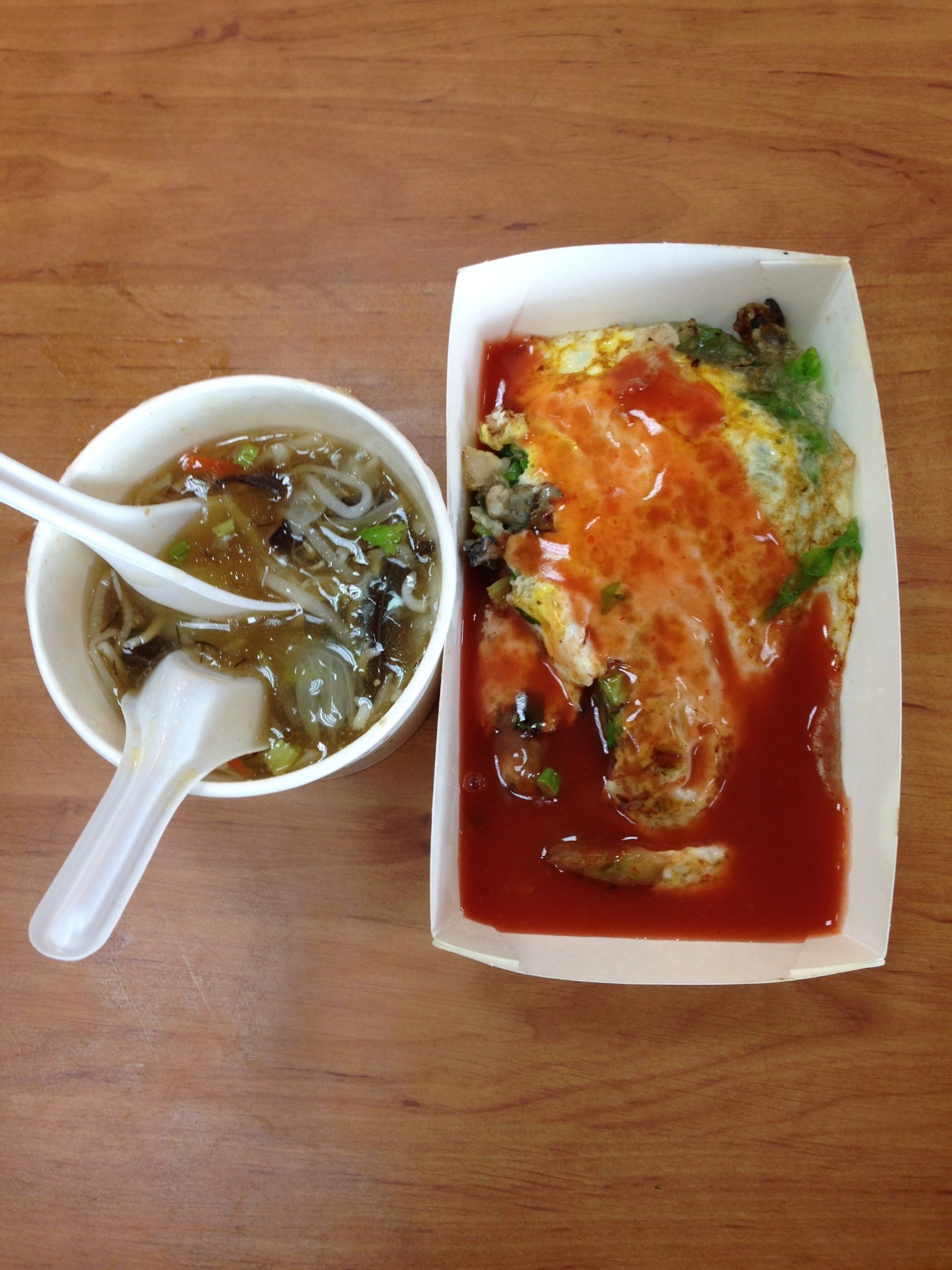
[0,451,299,620]
[29,651,271,961]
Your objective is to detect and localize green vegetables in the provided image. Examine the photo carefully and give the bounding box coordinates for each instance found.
[167,442,407,780]
[449,301,862,792]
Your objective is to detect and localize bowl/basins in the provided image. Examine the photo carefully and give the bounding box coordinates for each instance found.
[25,373,461,799]
